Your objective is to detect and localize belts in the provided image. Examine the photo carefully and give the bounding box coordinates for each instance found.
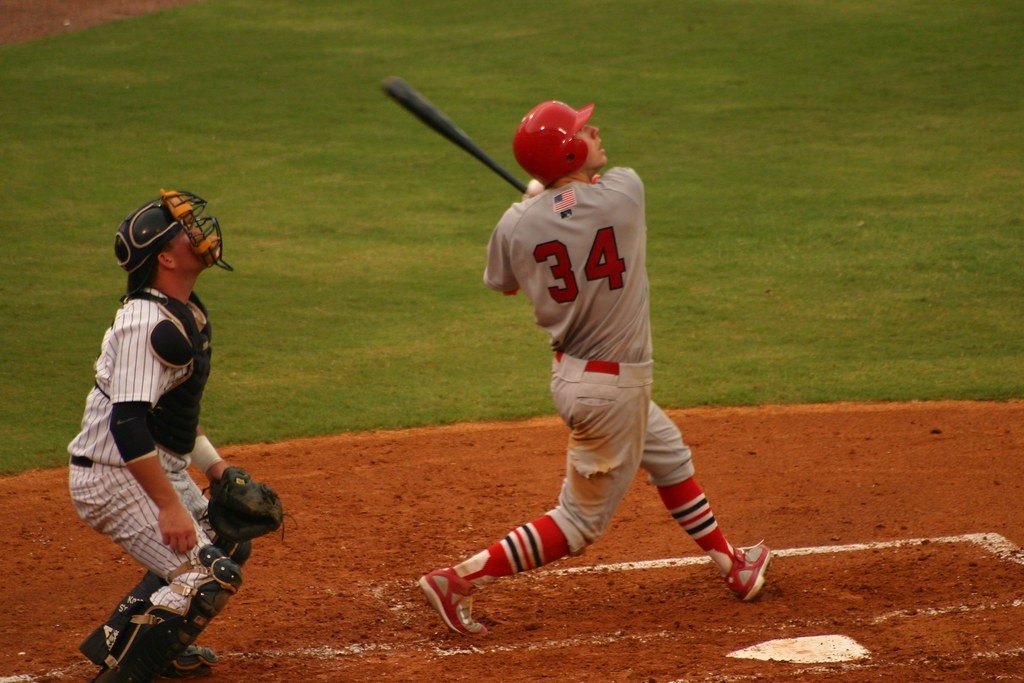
[554,351,620,375]
[70,456,92,467]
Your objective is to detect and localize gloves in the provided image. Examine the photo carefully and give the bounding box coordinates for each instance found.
[520,179,545,202]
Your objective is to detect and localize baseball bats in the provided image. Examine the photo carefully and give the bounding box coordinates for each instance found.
[379,75,528,195]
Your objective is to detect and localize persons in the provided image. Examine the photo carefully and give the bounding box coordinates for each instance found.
[66,190,283,683]
[420,99,769,638]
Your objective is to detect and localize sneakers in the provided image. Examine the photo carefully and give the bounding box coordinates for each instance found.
[418,568,489,638]
[720,539,771,601]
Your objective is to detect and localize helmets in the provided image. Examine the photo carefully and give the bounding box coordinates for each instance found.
[512,100,595,188]
[114,203,183,295]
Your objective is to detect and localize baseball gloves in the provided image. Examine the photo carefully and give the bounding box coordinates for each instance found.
[206,465,282,538]
